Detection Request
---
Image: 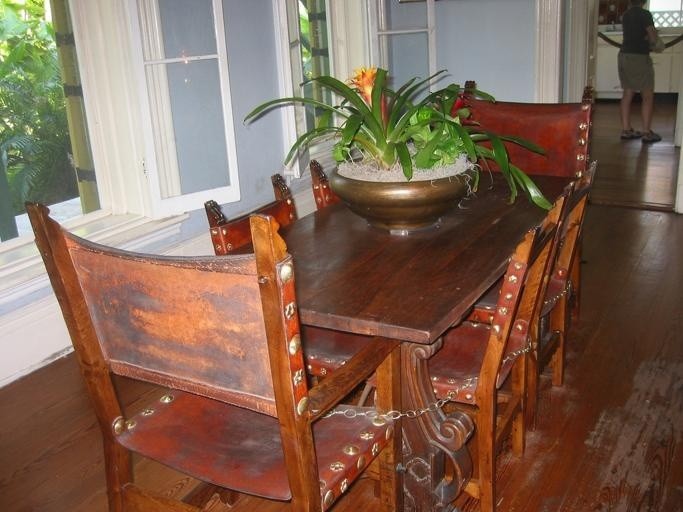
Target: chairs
[457,80,596,178]
[203,174,373,389]
[308,160,341,209]
[466,158,601,388]
[24,201,406,512]
[366,189,570,511]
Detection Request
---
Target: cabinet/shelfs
[594,24,683,94]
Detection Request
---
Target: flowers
[243,63,556,211]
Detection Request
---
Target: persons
[617,0,663,142]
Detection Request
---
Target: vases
[329,155,474,235]
[329,163,480,233]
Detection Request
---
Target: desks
[226,170,578,511]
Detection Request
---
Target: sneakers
[621,129,643,139]
[641,131,661,142]
